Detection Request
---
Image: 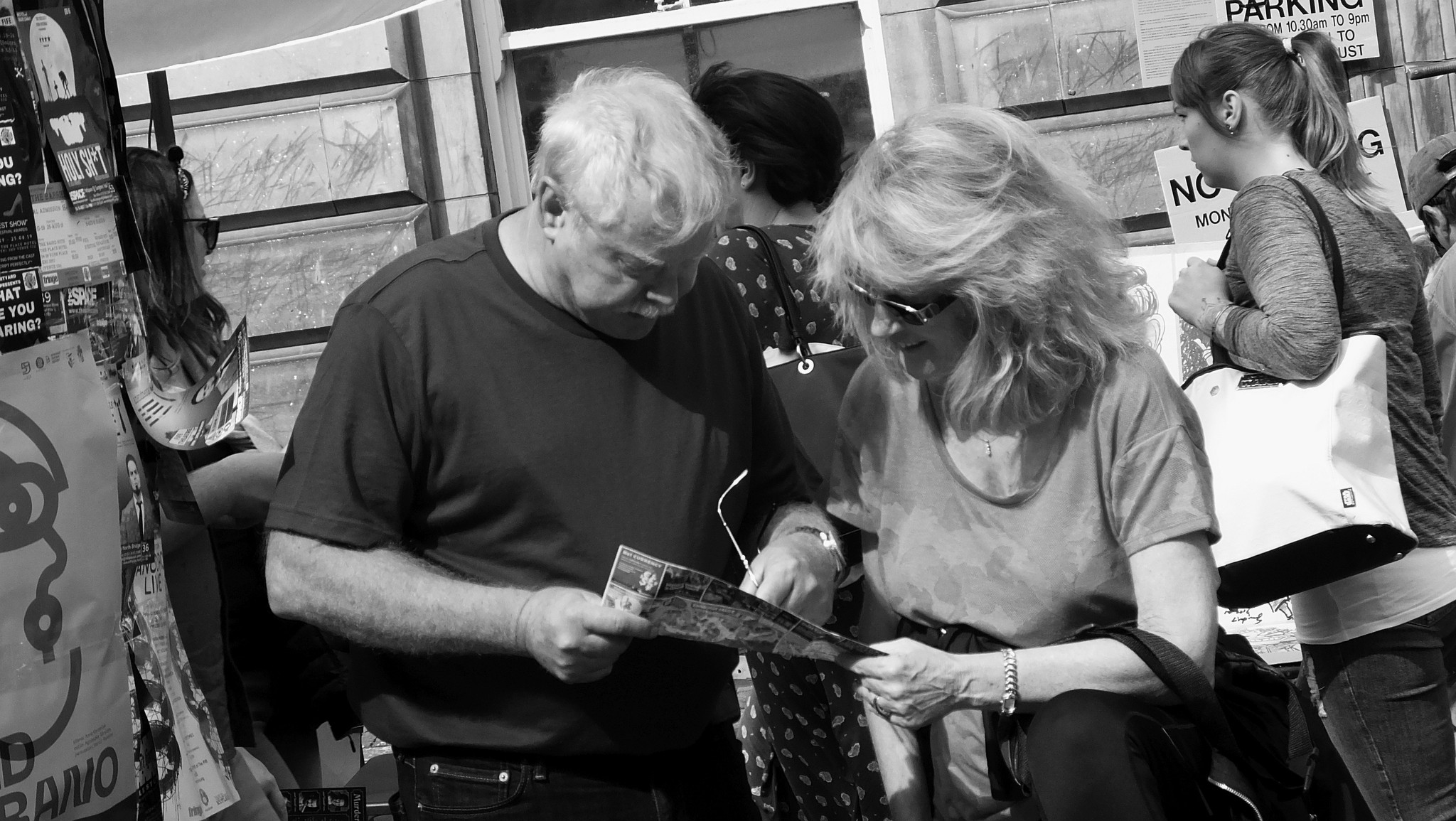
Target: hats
[1407,132,1456,219]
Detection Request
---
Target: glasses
[184,217,220,250]
[845,278,959,327]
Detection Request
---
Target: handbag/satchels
[1183,178,1418,597]
[726,222,870,521]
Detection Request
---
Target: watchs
[780,522,850,587]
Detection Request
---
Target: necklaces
[963,420,1003,458]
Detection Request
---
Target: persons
[689,62,903,821]
[1169,17,1456,821]
[805,104,1223,821]
[259,65,841,820]
[109,142,365,821]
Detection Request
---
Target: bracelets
[1000,646,1020,716]
[1209,302,1235,339]
